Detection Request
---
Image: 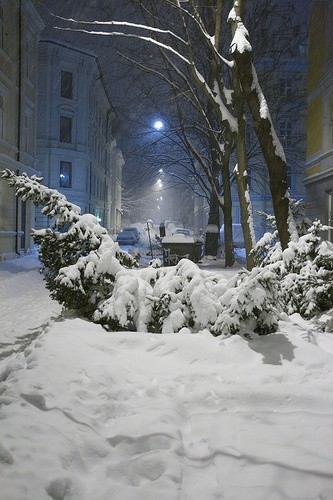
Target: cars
[173,228,193,236]
[116,227,140,245]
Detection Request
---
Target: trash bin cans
[194,240,203,264]
[204,231,219,260]
[161,242,195,267]
